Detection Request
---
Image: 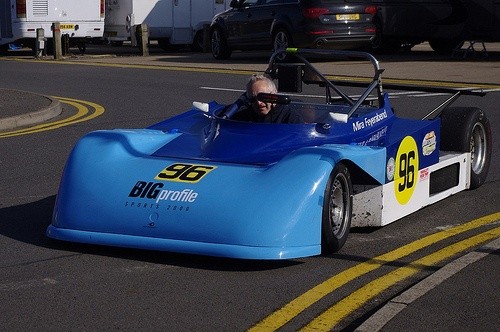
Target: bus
[0,0,107,52]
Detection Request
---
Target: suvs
[211,0,376,57]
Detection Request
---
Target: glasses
[247,95,259,103]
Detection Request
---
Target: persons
[220,73,307,125]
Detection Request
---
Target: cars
[49,47,492,265]
